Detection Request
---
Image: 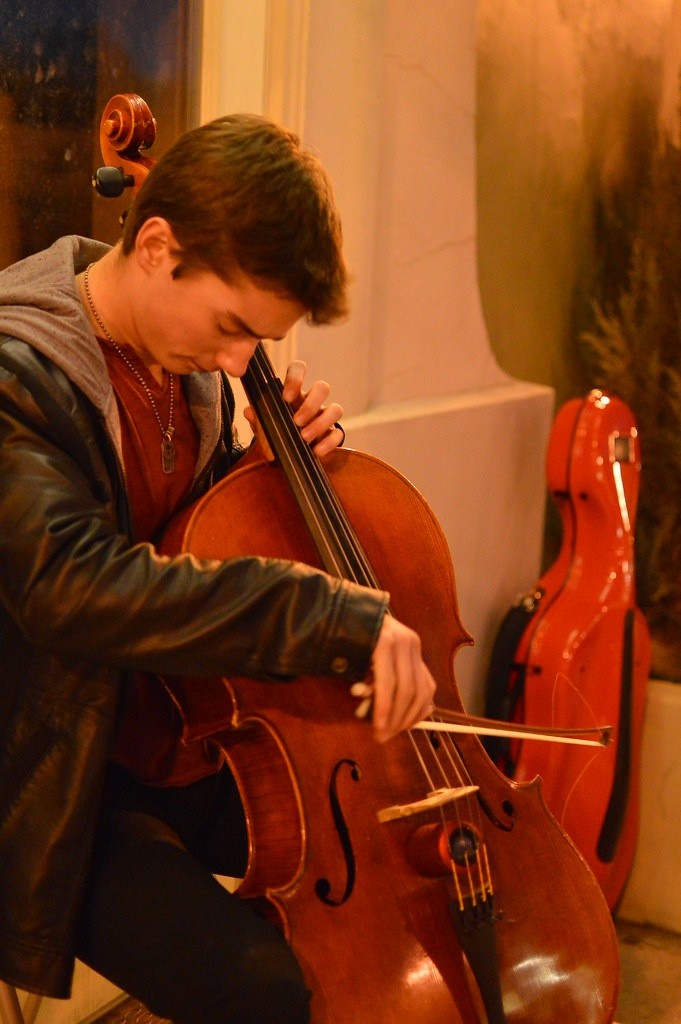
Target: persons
[0,114,437,1024]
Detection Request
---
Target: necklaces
[86,263,176,473]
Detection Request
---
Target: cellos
[92,87,627,1024]
